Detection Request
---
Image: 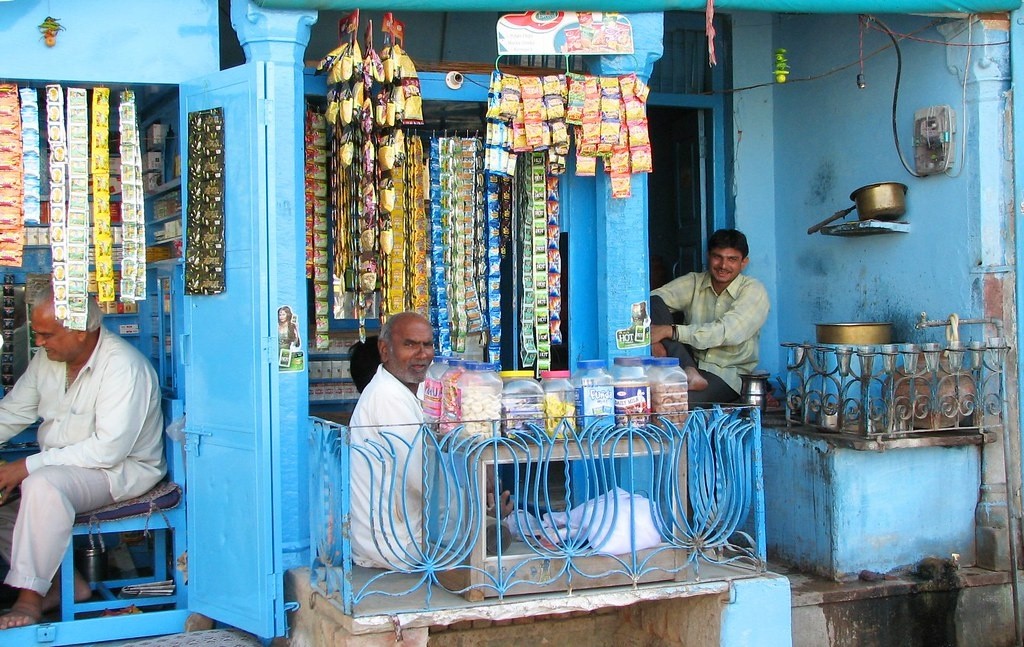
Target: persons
[278,306,300,352]
[343,312,513,572]
[649,228,771,411]
[631,303,648,330]
[350,335,560,520]
[0,283,167,629]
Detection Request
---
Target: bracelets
[670,323,678,342]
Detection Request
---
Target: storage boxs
[89,158,137,313]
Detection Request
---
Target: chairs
[60,398,188,621]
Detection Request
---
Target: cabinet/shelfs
[420,429,689,603]
[308,318,381,404]
[89,154,139,317]
[143,177,182,263]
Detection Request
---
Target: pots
[807,180,905,235]
[813,321,893,345]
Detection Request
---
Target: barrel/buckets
[74,543,109,582]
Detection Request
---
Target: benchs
[283,553,791,647]
